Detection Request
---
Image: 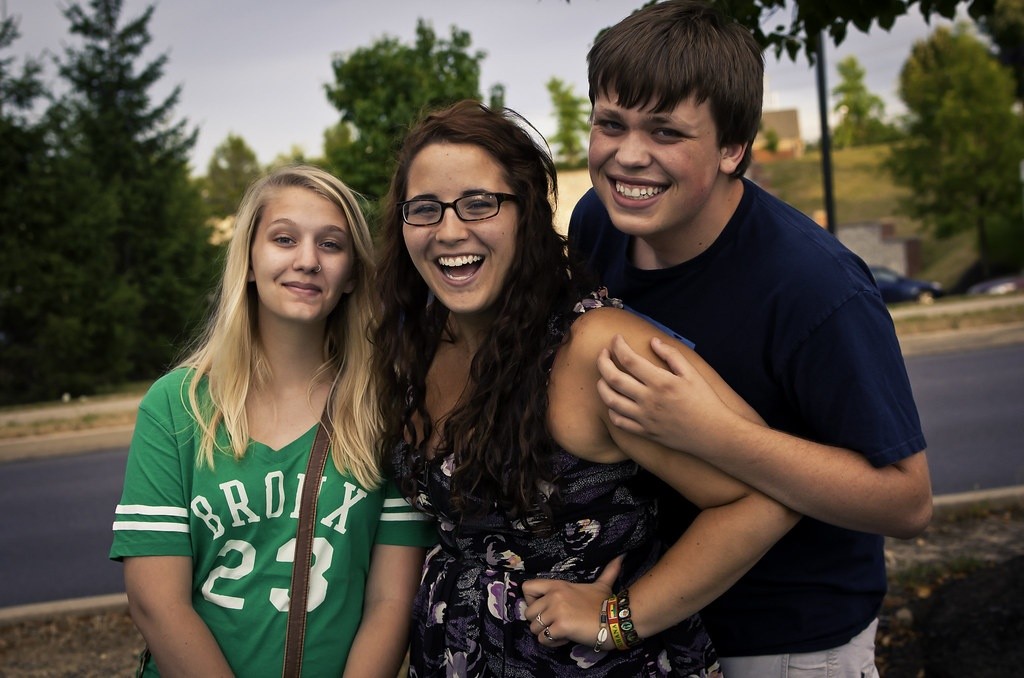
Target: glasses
[388,188,525,229]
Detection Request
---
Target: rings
[536,612,547,627]
[544,628,555,641]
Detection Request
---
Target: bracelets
[593,589,644,652]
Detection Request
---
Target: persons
[108,169,428,678]
[565,5,932,678]
[371,100,796,678]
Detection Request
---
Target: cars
[868,262,942,305]
